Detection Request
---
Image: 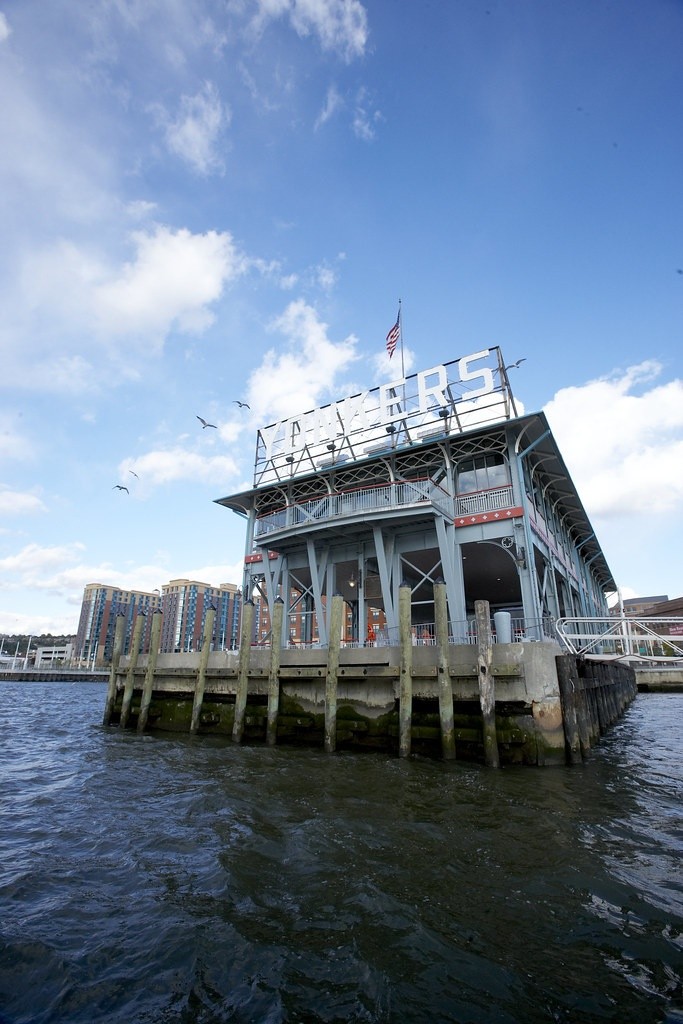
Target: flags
[385,299,401,360]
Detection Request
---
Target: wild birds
[504,358,527,372]
[111,485,129,495]
[231,400,251,410]
[195,415,218,429]
[127,469,140,481]
